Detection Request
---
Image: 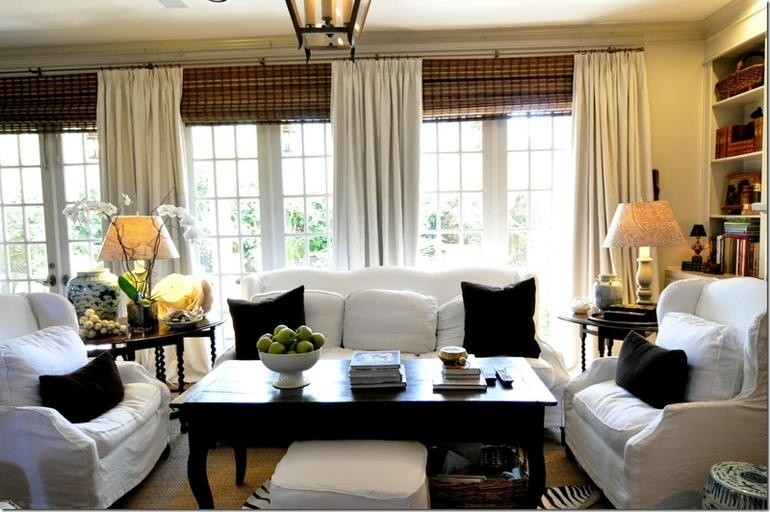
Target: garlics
[79,309,127,339]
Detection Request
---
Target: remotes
[493,365,514,383]
[481,366,497,381]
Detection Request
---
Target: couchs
[272,439,431,511]
[234,272,566,442]
[2,293,175,510]
[568,276,767,512]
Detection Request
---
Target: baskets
[714,48,764,101]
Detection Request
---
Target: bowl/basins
[480,446,514,475]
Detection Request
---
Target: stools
[701,461,768,511]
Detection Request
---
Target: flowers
[63,193,203,302]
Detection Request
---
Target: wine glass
[258,348,321,390]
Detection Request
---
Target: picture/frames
[720,172,762,211]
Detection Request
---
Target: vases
[66,269,119,337]
[127,301,155,331]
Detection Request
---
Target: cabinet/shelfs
[701,31,768,277]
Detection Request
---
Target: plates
[162,318,204,332]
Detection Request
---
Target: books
[433,354,487,390]
[715,218,761,276]
[348,350,407,389]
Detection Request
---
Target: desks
[79,318,226,436]
[557,306,659,377]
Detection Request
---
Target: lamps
[603,198,688,306]
[286,0,371,62]
[689,224,707,263]
[96,215,182,290]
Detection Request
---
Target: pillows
[1,324,86,405]
[435,293,465,353]
[253,290,345,350]
[460,276,539,359]
[342,288,437,353]
[39,350,121,421]
[225,285,307,359]
[614,324,685,410]
[657,304,739,401]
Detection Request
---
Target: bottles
[740,176,761,215]
